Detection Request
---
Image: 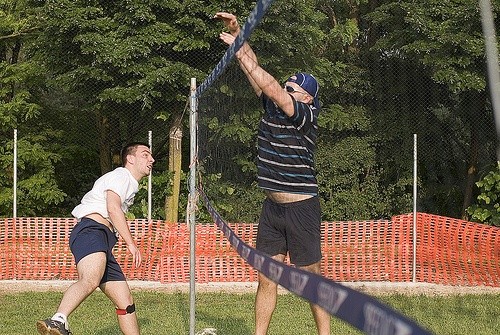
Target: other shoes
[37,318,73,335]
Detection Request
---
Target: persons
[213,12,331,335]
[37,142,155,335]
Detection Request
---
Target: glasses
[282,85,306,95]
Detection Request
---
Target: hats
[287,73,320,109]
